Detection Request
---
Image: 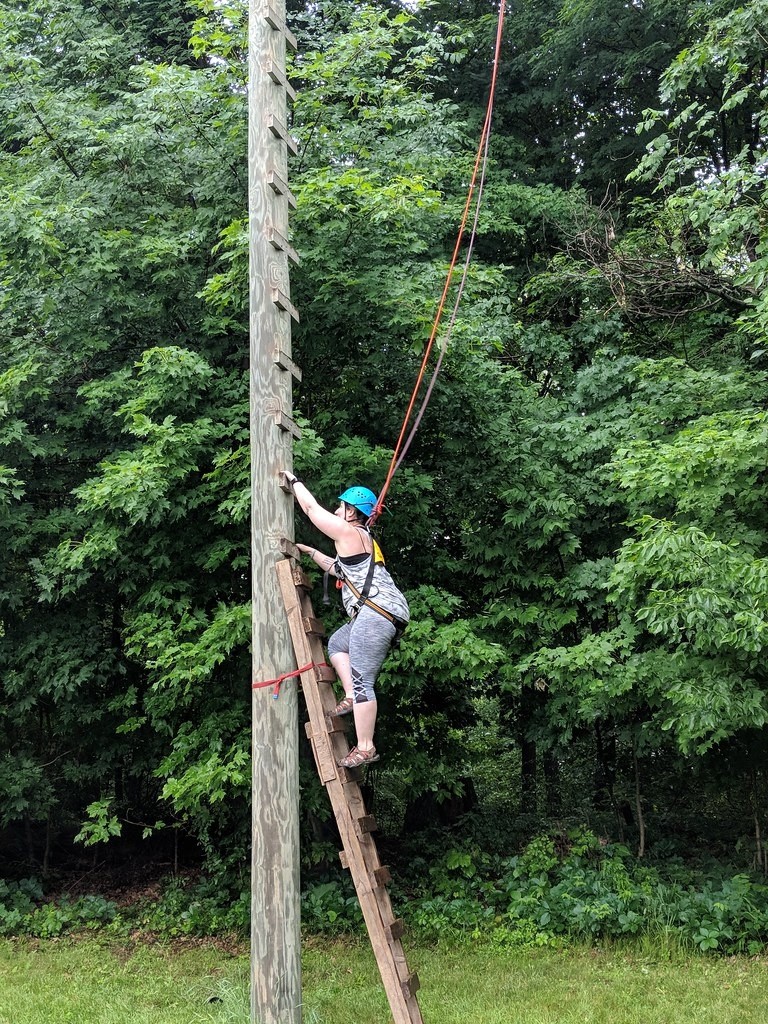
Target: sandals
[326,698,353,717]
[336,746,380,769]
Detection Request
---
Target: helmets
[338,486,377,518]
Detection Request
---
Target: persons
[279,471,410,768]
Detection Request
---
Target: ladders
[276,560,423,1024]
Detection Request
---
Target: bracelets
[311,550,316,560]
[291,478,304,485]
